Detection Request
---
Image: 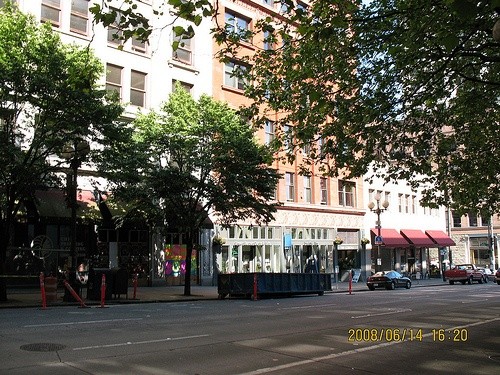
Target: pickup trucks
[446,263,486,285]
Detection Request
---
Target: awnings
[32,188,214,230]
[370,228,456,247]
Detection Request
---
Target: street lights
[369,193,389,273]
[57,141,90,303]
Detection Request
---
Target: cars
[367,270,411,290]
[427,265,438,278]
[494,268,500,285]
[478,269,489,283]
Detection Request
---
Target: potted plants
[334,240,343,244]
[214,237,225,255]
[361,239,371,245]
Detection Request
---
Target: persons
[76,261,88,285]
